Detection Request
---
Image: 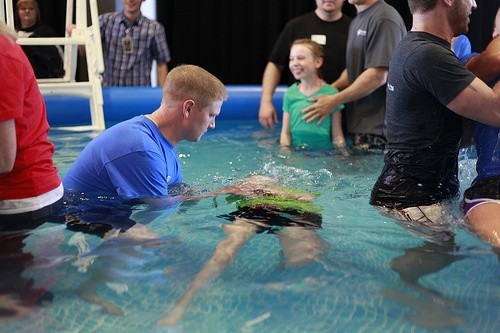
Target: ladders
[0,0,107,131]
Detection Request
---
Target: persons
[369,0,477,329]
[159,175,323,325]
[460,7,500,262]
[66,64,228,315]
[301,0,407,154]
[280,39,349,165]
[258,0,352,144]
[13,0,65,78]
[0,19,63,318]
[68,0,170,87]
